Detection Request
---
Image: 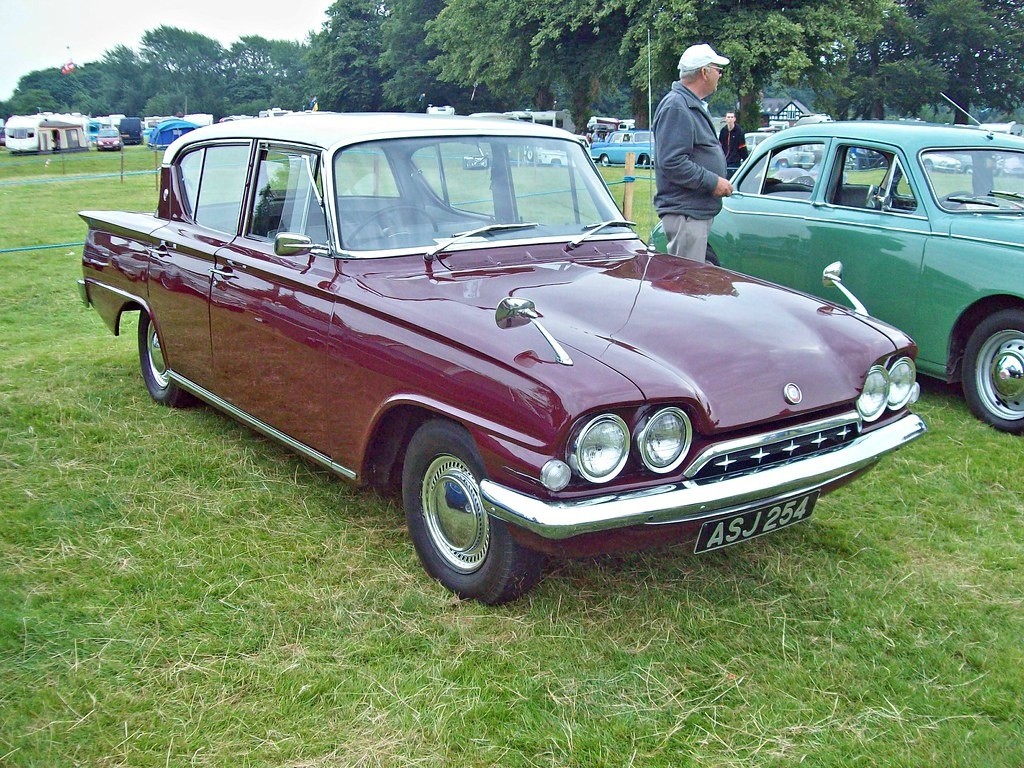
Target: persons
[580,129,610,144]
[651,43,734,263]
[719,110,749,181]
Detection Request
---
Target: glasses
[700,66,723,76]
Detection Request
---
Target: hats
[678,44,730,71]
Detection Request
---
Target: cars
[79,109,926,607]
[0,103,1024,172]
[647,116,1024,436]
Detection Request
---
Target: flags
[309,96,318,111]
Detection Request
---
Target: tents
[146,119,202,150]
